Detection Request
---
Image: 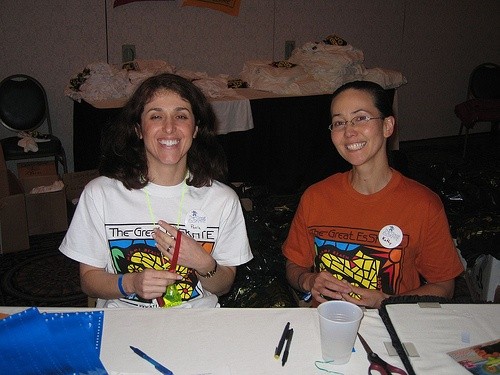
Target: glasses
[328,115,385,133]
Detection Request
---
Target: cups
[317,300,363,365]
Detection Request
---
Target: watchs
[196,261,218,278]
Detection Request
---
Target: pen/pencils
[281,329,293,366]
[130,346,172,375]
[274,322,290,358]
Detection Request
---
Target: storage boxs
[0,161,102,255]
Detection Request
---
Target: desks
[0,304,500,375]
[73,88,396,190]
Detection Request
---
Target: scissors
[357,332,408,375]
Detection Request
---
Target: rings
[166,246,172,253]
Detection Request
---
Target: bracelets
[118,274,132,297]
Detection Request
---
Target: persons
[58,73,253,308]
[282,80,463,308]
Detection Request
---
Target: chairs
[459,63,500,158]
[0,74,69,174]
